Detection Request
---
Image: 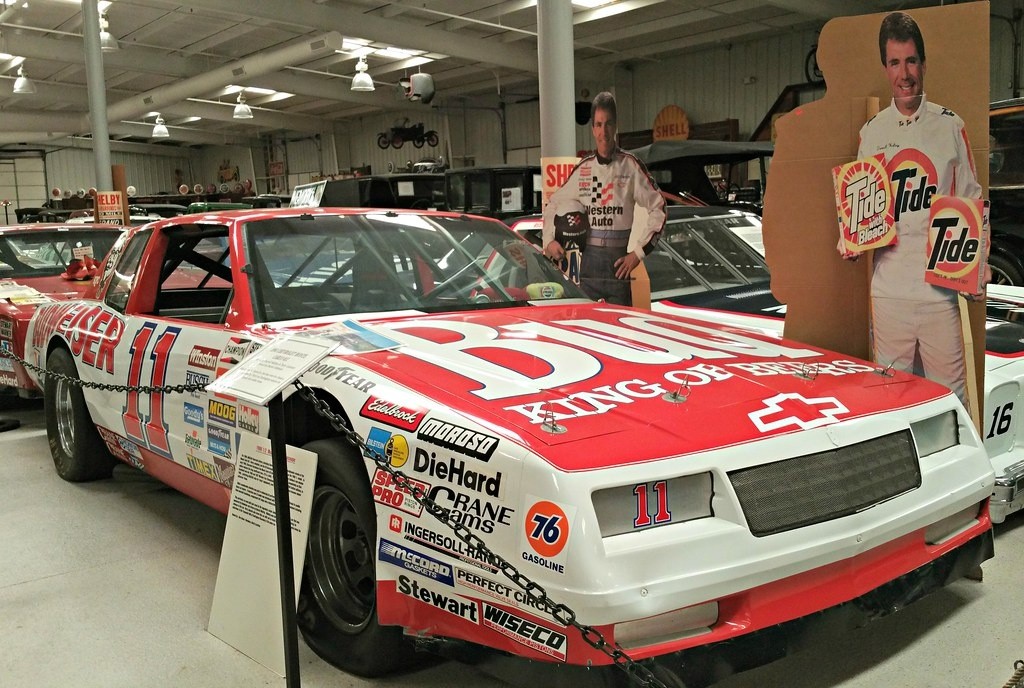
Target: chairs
[350,249,405,311]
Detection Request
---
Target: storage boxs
[832,152,897,256]
[924,194,991,296]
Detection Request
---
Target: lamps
[151,117,169,138]
[98,14,119,52]
[233,93,254,118]
[350,61,375,92]
[13,65,37,94]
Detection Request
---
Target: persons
[542,91,668,307]
[836,13,990,416]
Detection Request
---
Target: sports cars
[21,207,994,681]
[0,193,292,403]
[402,185,1024,556]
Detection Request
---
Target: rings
[624,268,628,271]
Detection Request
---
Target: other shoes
[60,259,90,281]
[84,254,100,278]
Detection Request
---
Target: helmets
[552,199,588,252]
